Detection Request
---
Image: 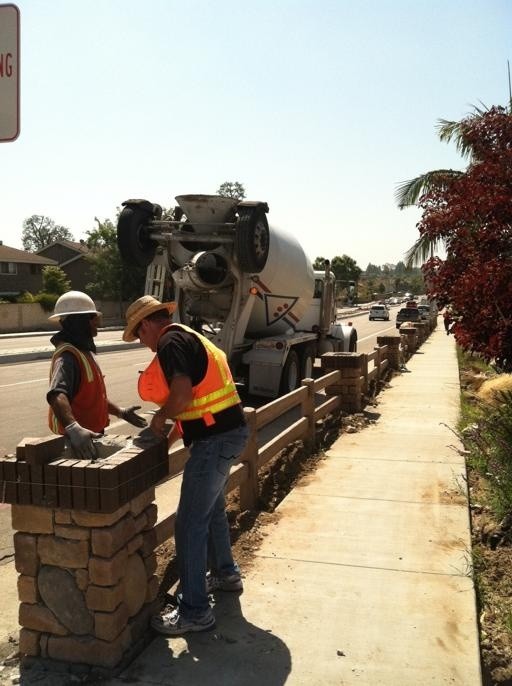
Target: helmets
[48,291,103,322]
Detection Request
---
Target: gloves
[65,420,101,460]
[119,406,148,428]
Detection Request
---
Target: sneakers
[151,608,216,635]
[205,571,243,594]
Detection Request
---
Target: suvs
[395,305,437,329]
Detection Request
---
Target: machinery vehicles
[114,192,361,405]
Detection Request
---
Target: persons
[120,294,250,638]
[443,310,450,331]
[41,288,150,460]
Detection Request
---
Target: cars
[378,290,434,317]
[368,303,390,322]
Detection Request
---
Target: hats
[122,295,178,342]
